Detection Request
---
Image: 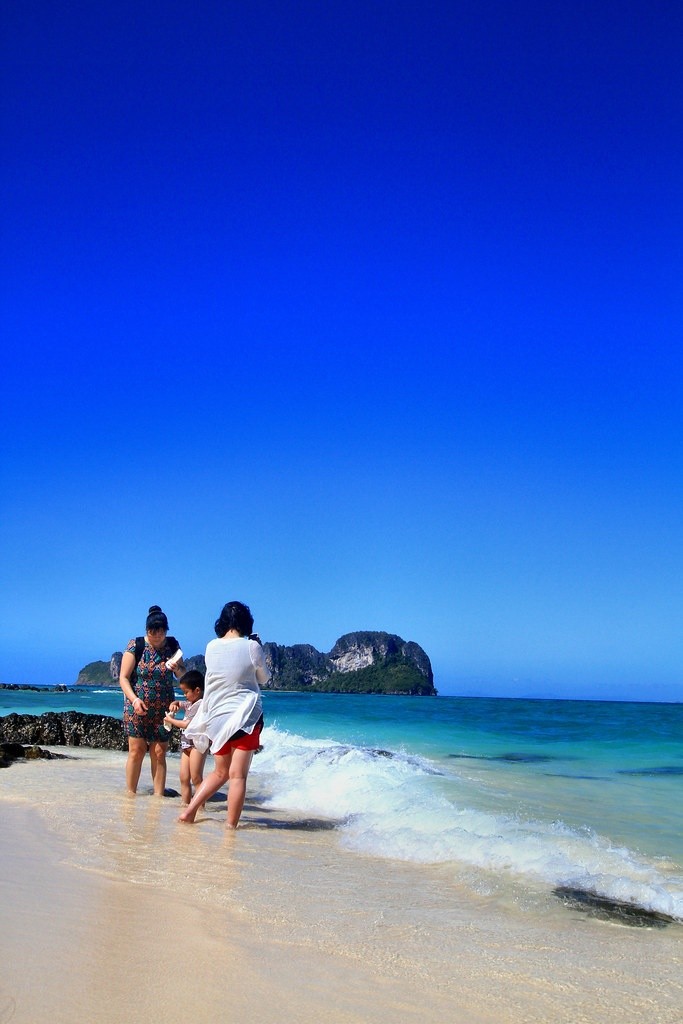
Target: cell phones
[248,633,258,641]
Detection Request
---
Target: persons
[163,670,208,810]
[119,605,186,797]
[178,601,272,828]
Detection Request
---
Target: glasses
[146,631,167,639]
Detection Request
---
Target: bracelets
[132,698,139,706]
